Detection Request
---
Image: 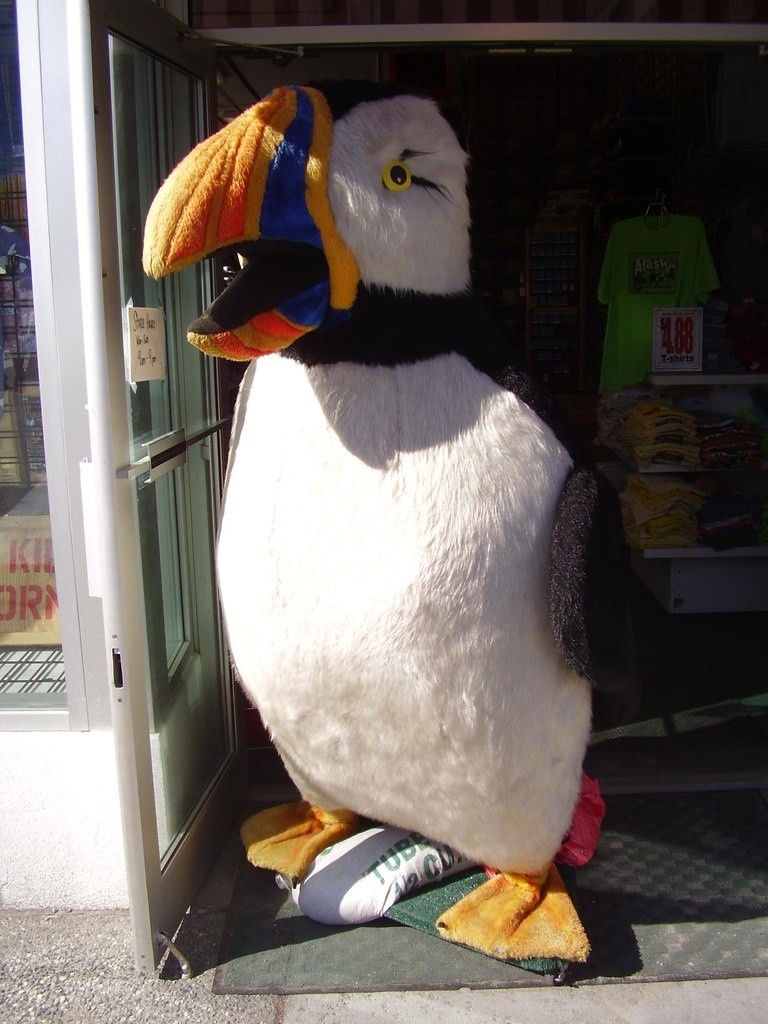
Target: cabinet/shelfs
[522,223,589,392]
[602,373,768,613]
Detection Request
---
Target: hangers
[641,187,670,218]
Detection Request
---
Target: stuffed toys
[141,74,718,966]
